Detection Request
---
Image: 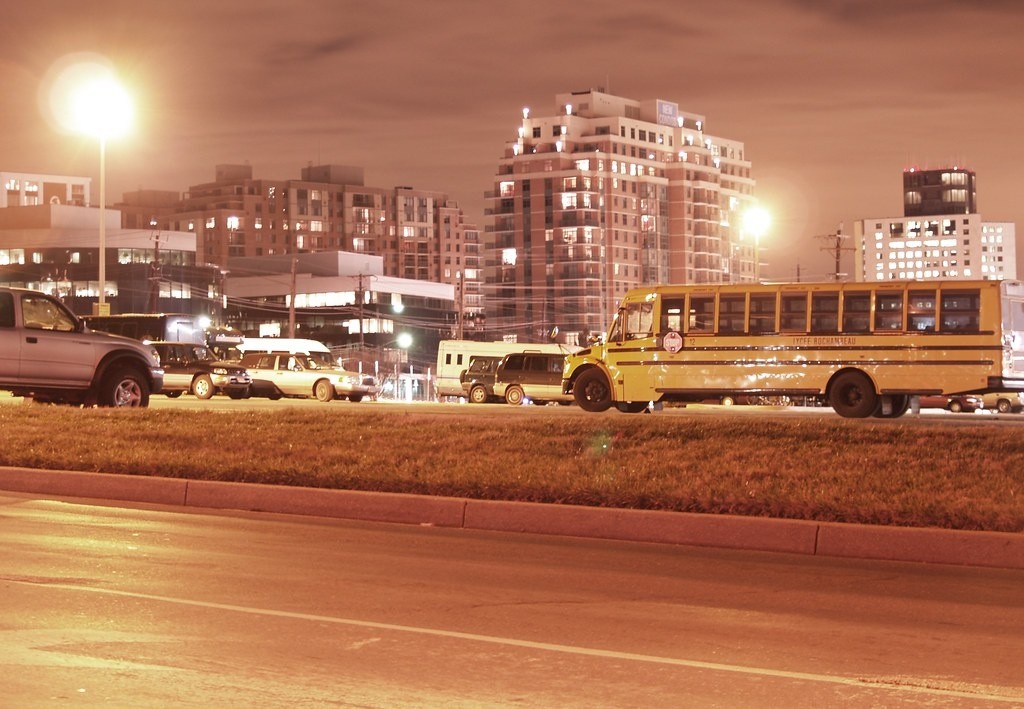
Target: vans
[492,353,580,406]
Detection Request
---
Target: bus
[560,278,1024,418]
[207,335,334,370]
[74,311,206,362]
[432,338,586,403]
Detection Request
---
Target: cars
[971,391,1024,414]
[908,394,979,413]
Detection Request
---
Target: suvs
[0,285,167,413]
[238,351,381,404]
[142,339,252,400]
[459,357,503,404]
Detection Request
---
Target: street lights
[81,86,132,310]
